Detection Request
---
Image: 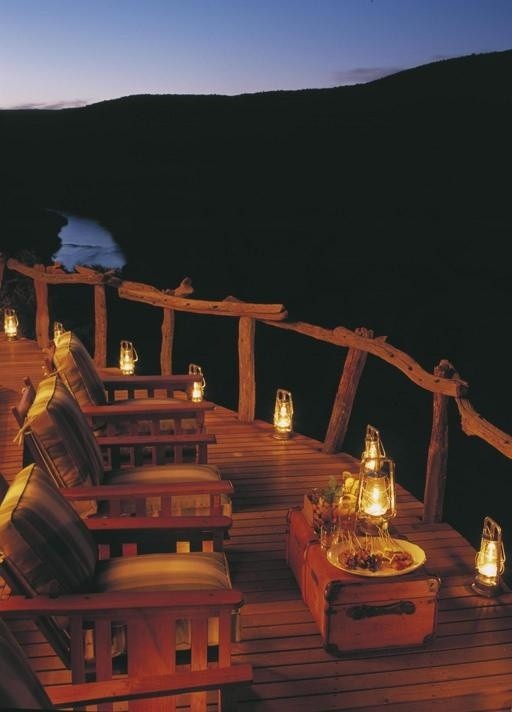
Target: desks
[286,505,443,657]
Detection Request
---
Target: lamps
[470,516,505,597]
[1,305,22,341]
[358,456,395,536]
[119,339,140,375]
[53,321,68,340]
[271,388,296,441]
[189,363,206,403]
[361,424,387,472]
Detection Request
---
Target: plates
[326,537,427,577]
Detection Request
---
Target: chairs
[0,618,252,711]
[11,371,235,548]
[0,463,244,678]
[39,330,216,463]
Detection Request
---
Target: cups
[320,523,340,555]
